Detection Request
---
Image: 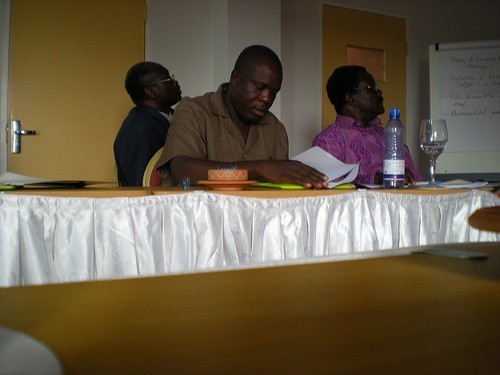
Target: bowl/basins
[207,169,248,180]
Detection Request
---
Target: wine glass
[419,119,448,189]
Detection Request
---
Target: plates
[196,180,257,191]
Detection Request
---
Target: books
[23,181,85,189]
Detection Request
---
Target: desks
[0,183,500,375]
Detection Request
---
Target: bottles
[382,108,406,189]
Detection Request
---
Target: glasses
[152,74,175,85]
[356,84,378,93]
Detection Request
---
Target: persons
[157,44,329,191]
[312,65,425,188]
[113,60,183,187]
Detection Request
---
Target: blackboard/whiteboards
[429,39,500,174]
